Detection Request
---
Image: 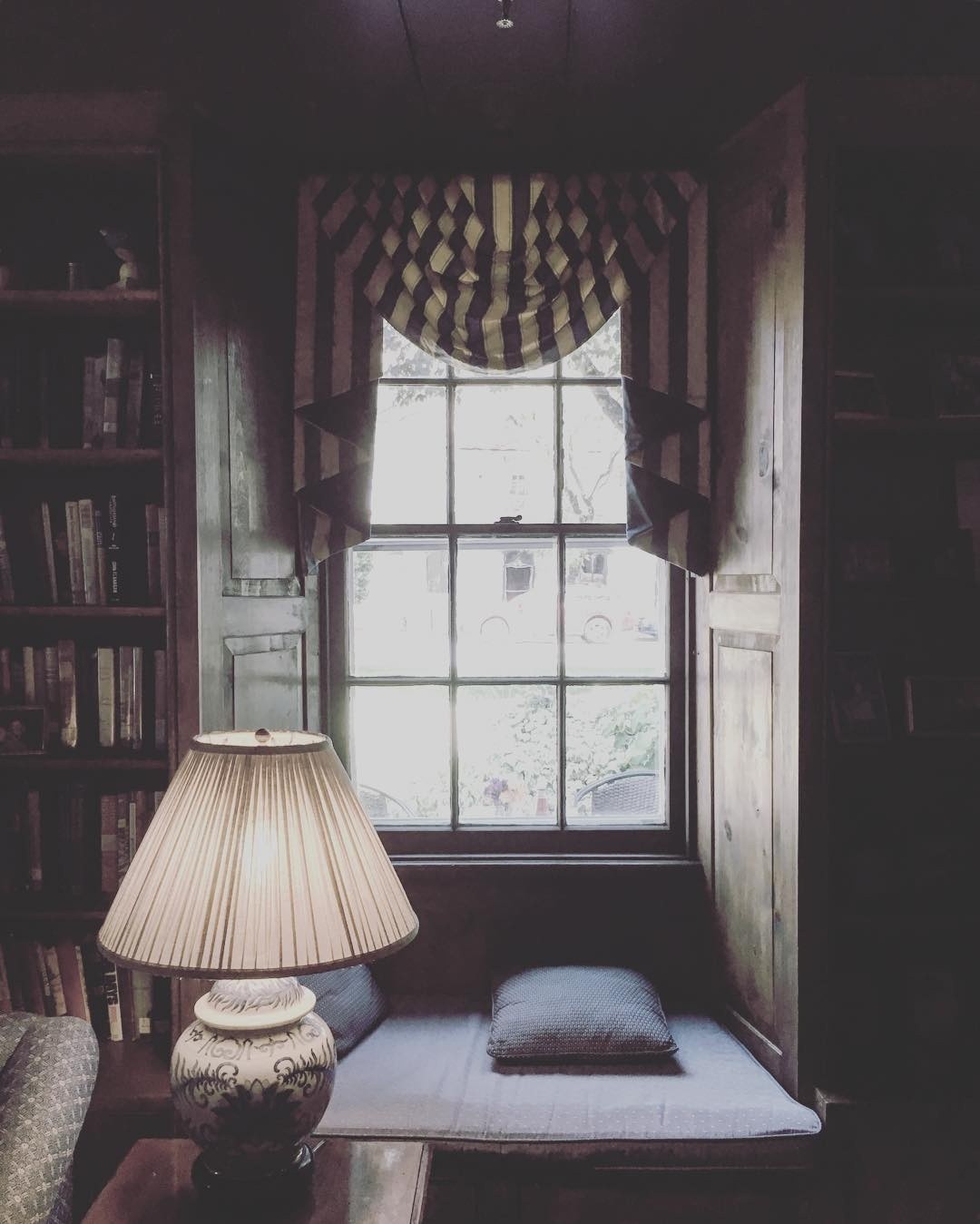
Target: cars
[454,584,634,645]
[638,612,664,641]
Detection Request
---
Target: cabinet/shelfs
[0,86,176,1119]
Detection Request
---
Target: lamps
[97,726,421,1224]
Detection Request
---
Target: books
[2,330,166,1049]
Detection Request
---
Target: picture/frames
[833,365,888,424]
[902,675,980,741]
[926,348,980,422]
[830,650,893,743]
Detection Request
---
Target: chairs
[360,782,415,820]
[574,769,659,821]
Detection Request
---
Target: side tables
[80,1136,432,1224]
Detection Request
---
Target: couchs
[0,1010,101,1224]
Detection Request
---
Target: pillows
[293,961,393,1069]
[484,962,679,1069]
